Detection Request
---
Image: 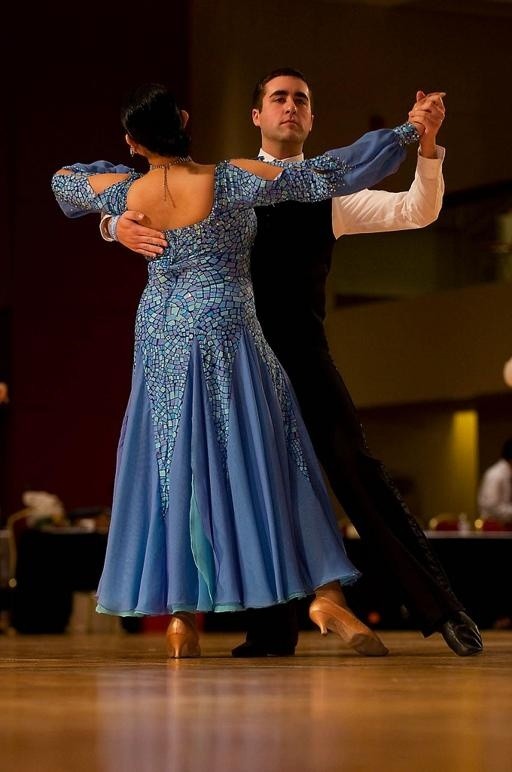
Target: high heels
[309,596,388,656]
[166,616,201,658]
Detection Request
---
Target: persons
[51,88,445,662]
[98,69,485,658]
[475,436,512,532]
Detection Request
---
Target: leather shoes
[231,642,295,657]
[440,609,483,657]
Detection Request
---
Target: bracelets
[108,214,120,243]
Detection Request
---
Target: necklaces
[148,155,193,172]
